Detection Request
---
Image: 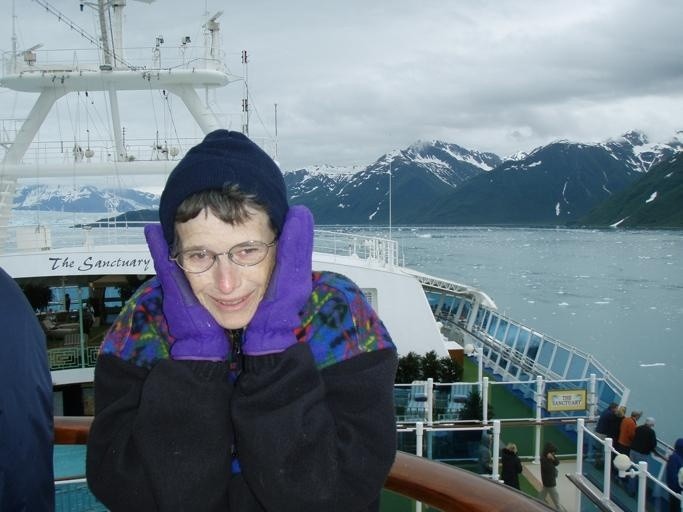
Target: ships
[1,0,683,511]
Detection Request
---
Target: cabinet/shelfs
[444,341,464,367]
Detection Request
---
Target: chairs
[407,380,472,412]
[42,320,80,338]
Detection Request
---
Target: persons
[537,441,562,511]
[84,129,399,512]
[626,416,657,499]
[665,438,683,512]
[607,404,628,473]
[614,409,642,487]
[475,433,494,475]
[593,401,619,471]
[501,442,524,489]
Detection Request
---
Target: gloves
[240,206,314,357]
[145,223,230,359]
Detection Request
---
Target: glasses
[168,237,278,274]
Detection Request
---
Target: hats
[159,129,289,246]
